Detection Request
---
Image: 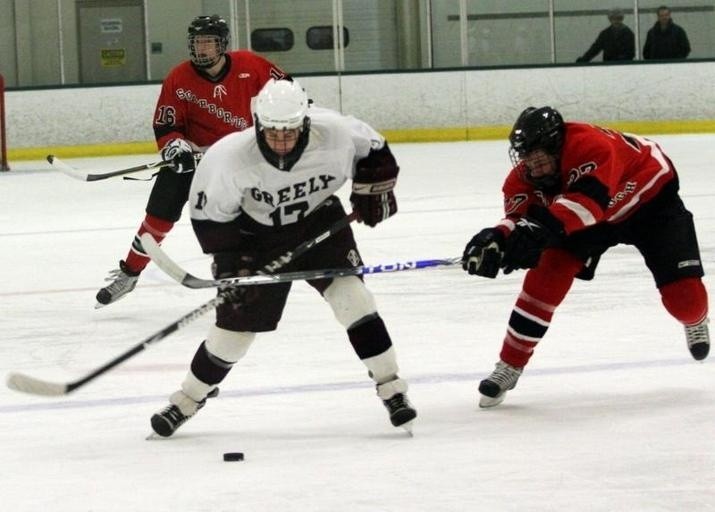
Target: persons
[150,79,421,438]
[93,14,315,305]
[464,106,712,400]
[576,8,635,64]
[642,5,692,60]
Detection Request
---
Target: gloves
[350,168,565,277]
[162,137,198,174]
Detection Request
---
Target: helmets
[255,78,309,157]
[508,106,564,181]
[187,14,231,67]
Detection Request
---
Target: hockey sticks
[45,153,173,182]
[140,231,464,289]
[7,213,355,396]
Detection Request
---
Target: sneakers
[376,378,416,426]
[96,260,139,304]
[479,359,523,395]
[684,320,709,361]
[151,391,205,438]
